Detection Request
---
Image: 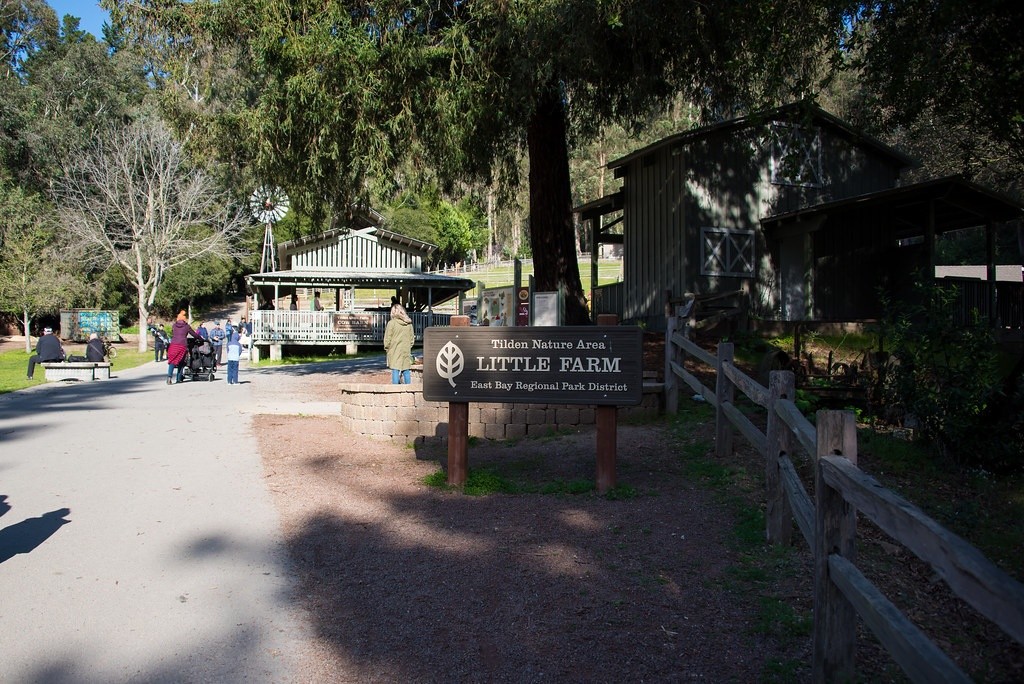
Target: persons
[86,333,107,362]
[227,332,242,385]
[258,297,274,310]
[197,322,208,341]
[155,324,169,362]
[26,326,64,380]
[226,318,258,348]
[482,297,499,316]
[99,331,111,354]
[167,314,204,385]
[391,296,401,308]
[290,294,298,310]
[384,304,414,383]
[210,322,225,366]
[314,292,324,311]
[33,322,59,337]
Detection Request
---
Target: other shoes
[167,377,171,385]
[228,383,231,385]
[178,381,182,383]
[27,376,33,380]
[234,383,240,385]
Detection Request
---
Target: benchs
[41,362,98,381]
[72,362,113,380]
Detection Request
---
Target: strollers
[179,339,220,382]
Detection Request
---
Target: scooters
[103,335,119,359]
